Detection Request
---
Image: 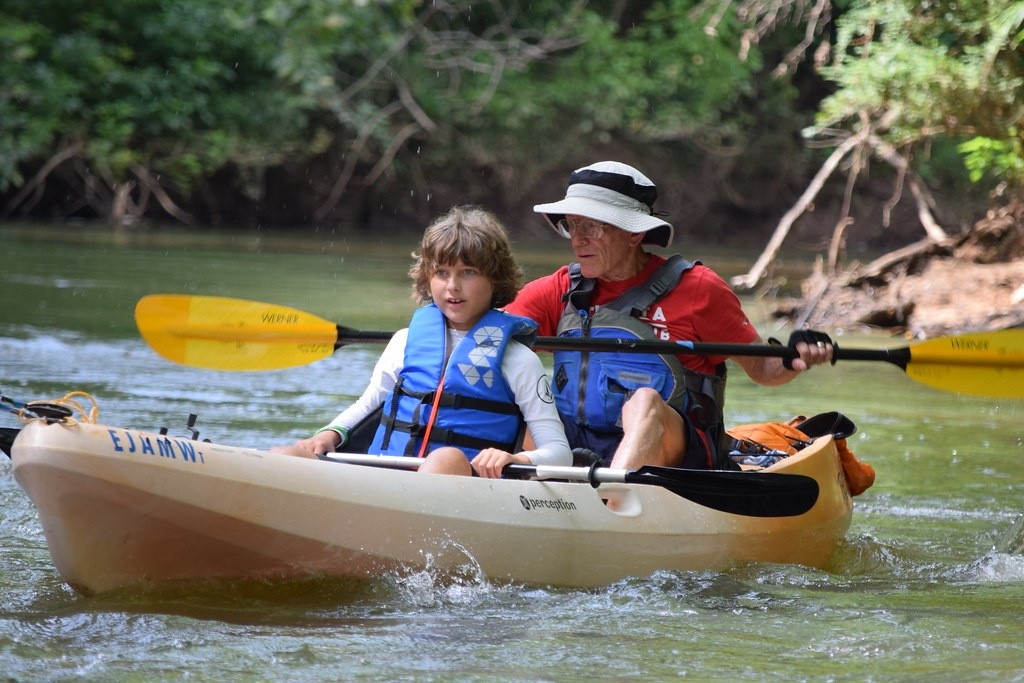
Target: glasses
[554,217,620,240]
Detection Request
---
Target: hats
[532,160,675,252]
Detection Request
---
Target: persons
[268,207,573,480]
[496,161,834,512]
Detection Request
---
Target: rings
[817,341,825,347]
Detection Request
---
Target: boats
[9,417,857,604]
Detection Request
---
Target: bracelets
[314,426,348,448]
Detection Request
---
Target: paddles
[0,425,821,519]
[132,291,1024,413]
